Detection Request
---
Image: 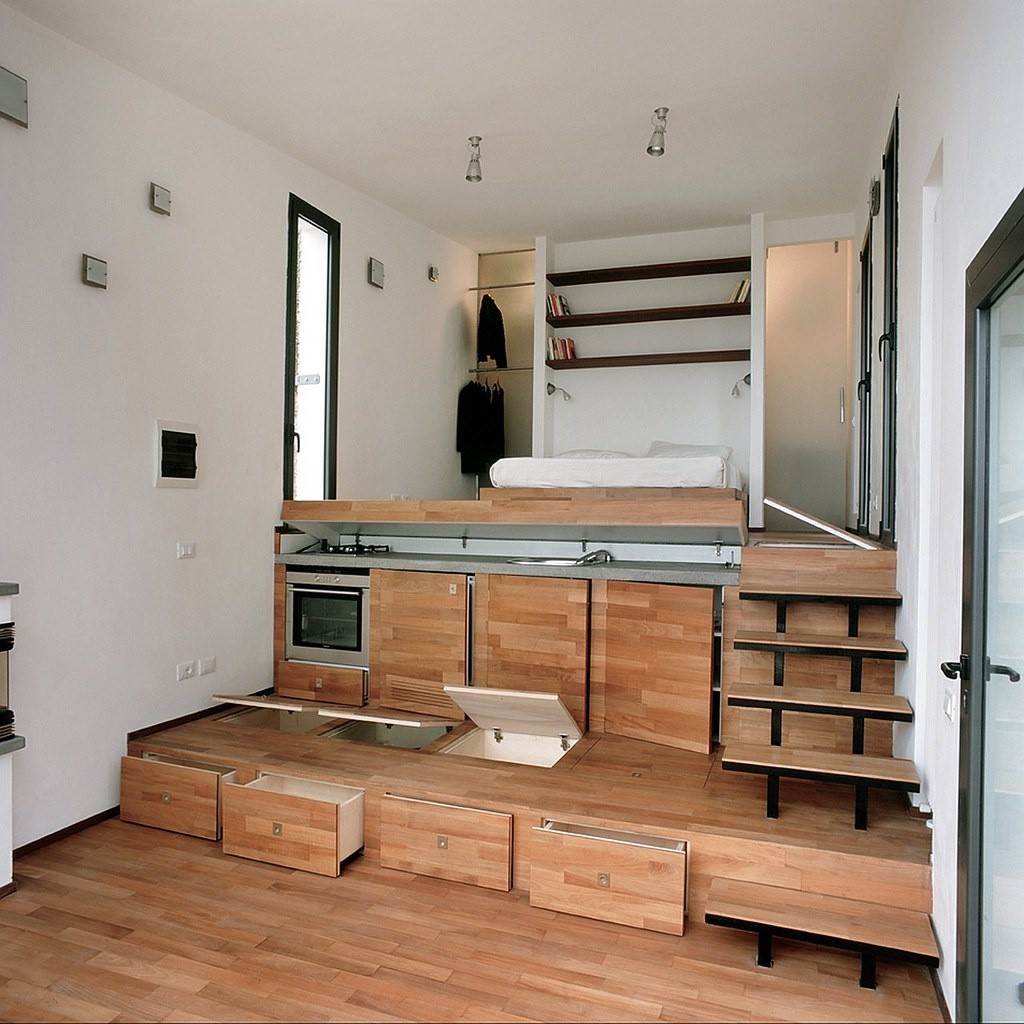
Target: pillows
[553,449,634,457]
[644,442,733,460]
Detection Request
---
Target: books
[730,279,750,303]
[547,293,570,317]
[547,336,576,360]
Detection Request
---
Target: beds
[489,457,746,491]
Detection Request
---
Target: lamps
[732,374,750,395]
[647,108,669,155]
[547,383,571,401]
[465,136,483,182]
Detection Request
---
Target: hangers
[487,286,495,299]
[471,368,501,389]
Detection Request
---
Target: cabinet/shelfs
[121,565,725,935]
[467,282,536,373]
[545,254,751,369]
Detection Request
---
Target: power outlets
[177,660,194,681]
